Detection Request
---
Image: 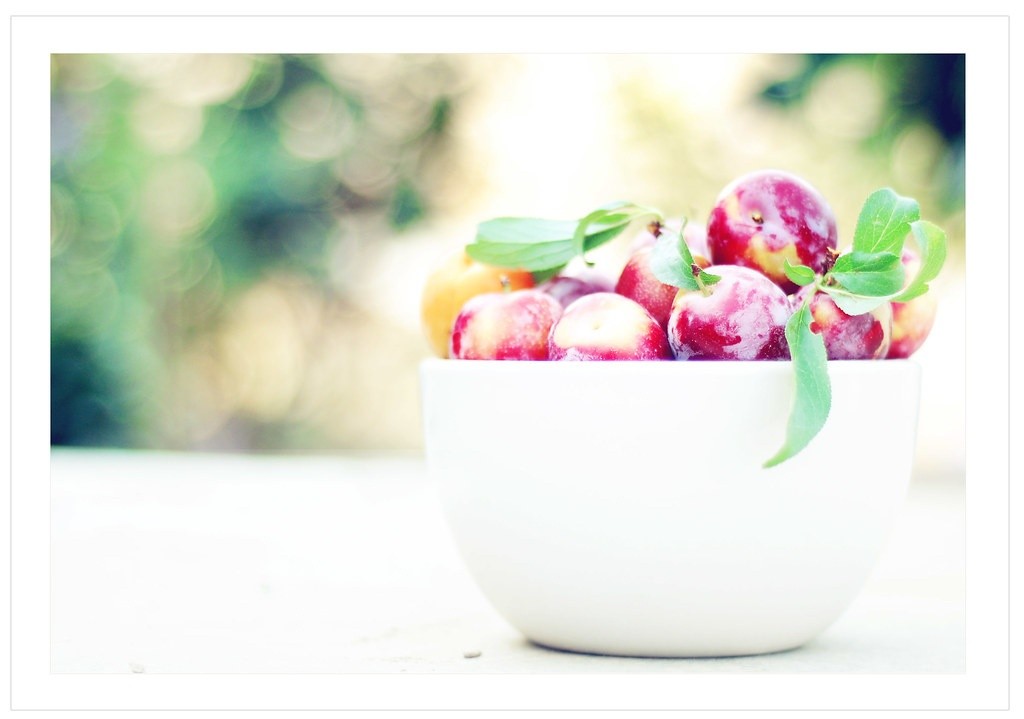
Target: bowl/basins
[421,360,915,660]
